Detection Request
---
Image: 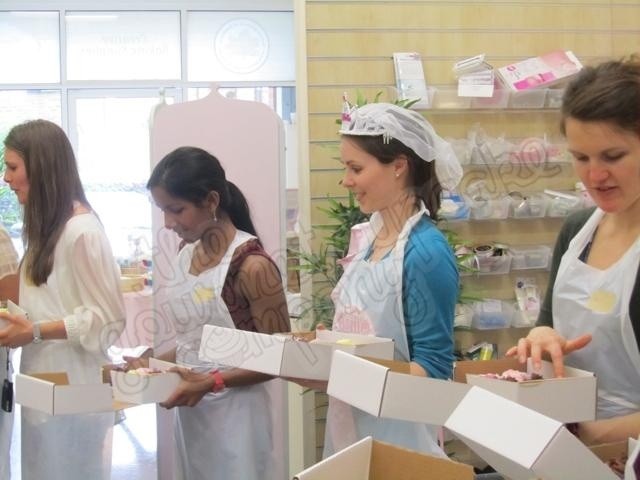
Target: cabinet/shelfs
[389,86,597,330]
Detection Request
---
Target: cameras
[2,379,14,412]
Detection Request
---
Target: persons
[269,100,461,459]
[119,145,293,479]
[1,116,126,480]
[0,226,24,480]
[500,57,640,480]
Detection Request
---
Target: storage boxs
[0,299,620,480]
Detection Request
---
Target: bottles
[479,343,493,361]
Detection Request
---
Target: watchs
[33,322,44,345]
[208,368,225,395]
